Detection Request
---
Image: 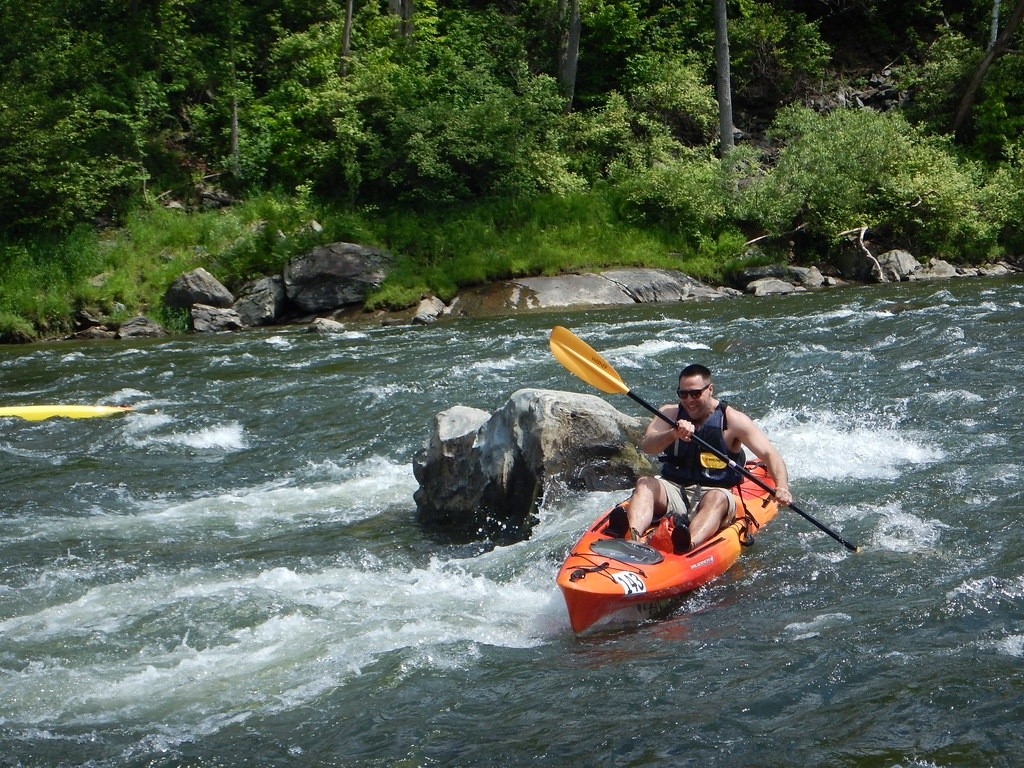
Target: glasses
[676,383,709,399]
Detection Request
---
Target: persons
[609,364,794,555]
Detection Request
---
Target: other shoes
[601,506,630,540]
[671,524,691,555]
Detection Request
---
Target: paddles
[548,324,863,551]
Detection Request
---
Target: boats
[556,455,780,639]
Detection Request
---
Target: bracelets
[774,487,792,497]
